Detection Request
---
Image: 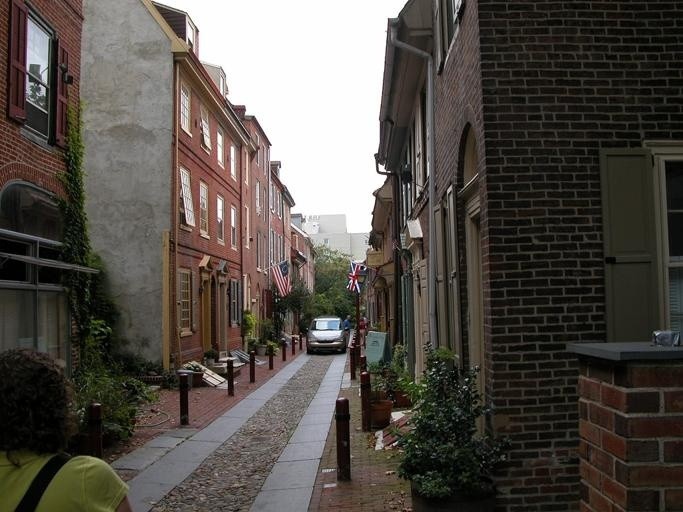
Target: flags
[271,261,291,297]
[345,261,367,294]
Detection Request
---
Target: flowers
[183,360,207,372]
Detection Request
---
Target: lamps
[372,258,394,289]
[375,154,412,182]
[57,61,74,84]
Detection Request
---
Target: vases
[194,373,203,386]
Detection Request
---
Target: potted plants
[256,336,267,356]
[370,345,412,427]
[205,349,218,367]
[386,343,512,512]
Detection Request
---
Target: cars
[305,315,349,354]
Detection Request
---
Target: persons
[0,346,133,511]
[342,314,351,348]
[358,318,367,346]
[359,311,368,324]
